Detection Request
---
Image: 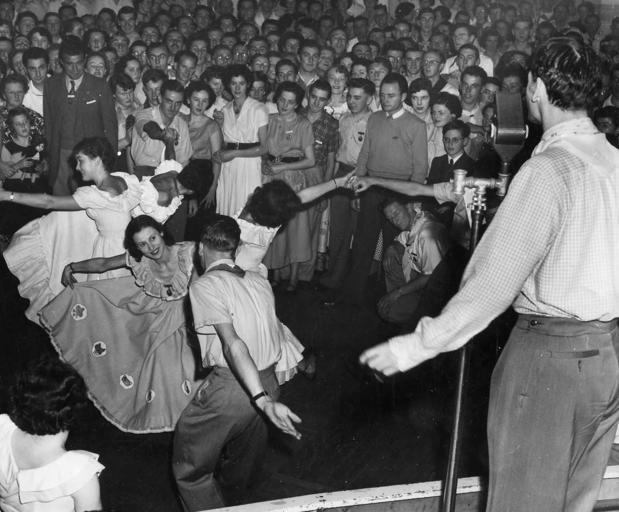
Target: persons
[141,25,160,46]
[147,41,169,71]
[0,19,13,41]
[337,53,358,72]
[182,79,224,240]
[420,50,446,92]
[218,14,237,33]
[1,357,106,512]
[478,76,500,106]
[423,121,479,214]
[44,13,62,42]
[118,6,138,33]
[205,28,224,50]
[1,73,44,140]
[327,28,346,54]
[366,59,391,113]
[367,27,385,46]
[332,0,392,37]
[111,34,130,55]
[236,21,259,42]
[592,18,619,149]
[427,93,463,173]
[136,0,195,18]
[442,25,494,77]
[130,41,148,67]
[0,38,15,67]
[392,0,435,47]
[58,6,77,21]
[43,35,118,196]
[429,0,455,50]
[221,33,238,47]
[348,73,428,305]
[153,12,172,36]
[257,80,315,293]
[319,47,336,71]
[0,1,16,20]
[296,40,319,87]
[414,227,473,323]
[501,70,525,93]
[101,46,119,73]
[16,11,37,32]
[46,42,66,73]
[85,51,111,79]
[279,32,304,56]
[248,36,269,55]
[267,52,282,79]
[1,136,146,282]
[2,128,213,334]
[360,37,619,512]
[250,72,279,114]
[321,79,376,293]
[453,0,532,25]
[30,27,52,49]
[296,0,333,39]
[175,16,194,39]
[323,64,351,121]
[351,43,373,61]
[118,55,141,84]
[265,32,280,51]
[459,66,485,125]
[251,54,269,73]
[274,58,297,84]
[232,41,249,65]
[350,175,478,227]
[109,74,141,172]
[4,109,48,193]
[237,0,255,20]
[173,50,197,83]
[346,16,369,53]
[532,0,600,40]
[12,35,31,49]
[131,79,193,180]
[192,6,211,29]
[233,167,360,279]
[408,79,435,138]
[62,18,85,40]
[80,14,98,32]
[378,198,448,323]
[213,45,233,66]
[351,59,369,79]
[255,0,296,34]
[10,48,28,74]
[38,216,214,433]
[166,30,187,53]
[369,41,380,60]
[385,43,404,73]
[299,80,340,289]
[21,47,55,118]
[214,65,269,216]
[186,34,212,80]
[495,15,532,70]
[171,214,301,512]
[85,30,108,50]
[401,48,424,85]
[98,8,116,31]
[220,0,234,15]
[446,44,480,89]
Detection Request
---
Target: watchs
[249,391,269,404]
[10,191,14,201]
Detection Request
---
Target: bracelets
[332,178,338,190]
[69,262,76,274]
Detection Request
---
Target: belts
[226,143,261,150]
[266,153,298,163]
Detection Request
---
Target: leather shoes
[271,281,282,289]
[286,284,300,295]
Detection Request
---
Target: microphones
[490,88,530,161]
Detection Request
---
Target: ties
[68,81,77,95]
[449,158,455,164]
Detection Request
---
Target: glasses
[235,53,249,57]
[130,51,147,56]
[150,54,169,61]
[423,61,441,67]
[301,52,321,61]
[389,56,402,62]
[217,55,231,59]
